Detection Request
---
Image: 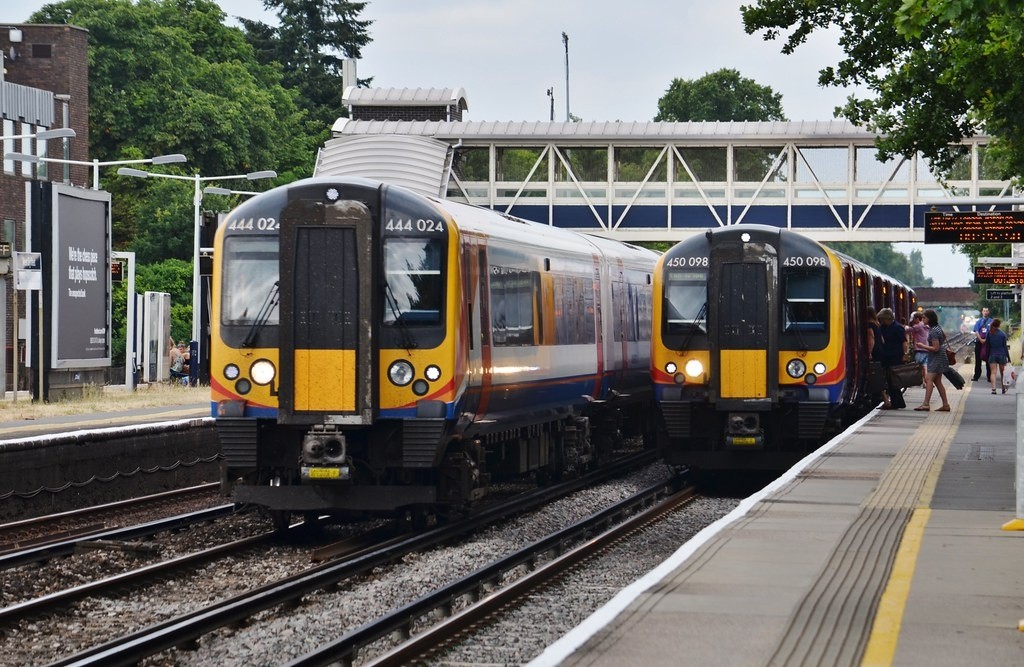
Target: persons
[167,338,190,381]
[864,306,1011,413]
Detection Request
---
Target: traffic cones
[117,166,278,389]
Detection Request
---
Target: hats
[876,307,894,319]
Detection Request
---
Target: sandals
[934,406,950,411]
[914,405,929,411]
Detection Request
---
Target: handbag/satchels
[1002,362,1015,386]
[980,343,988,361]
[946,349,956,365]
[890,360,922,392]
[985,332,990,355]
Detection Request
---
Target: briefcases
[857,353,889,393]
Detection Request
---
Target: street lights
[5,147,187,191]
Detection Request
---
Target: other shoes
[991,388,996,394]
[923,382,935,387]
[891,400,906,409]
[883,401,891,409]
[988,378,991,382]
[1002,387,1008,394]
[971,377,978,381]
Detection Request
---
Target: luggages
[943,367,965,389]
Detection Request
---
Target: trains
[644,217,918,490]
[197,173,705,537]
